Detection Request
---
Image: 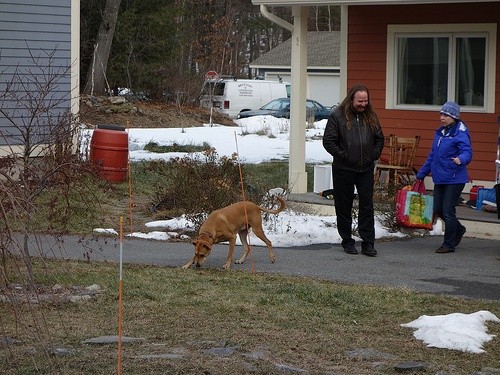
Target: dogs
[182,195,288,269]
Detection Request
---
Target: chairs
[374,134,421,192]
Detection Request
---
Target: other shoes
[361,243,377,256]
[454,225,466,247]
[435,243,455,253]
[344,244,357,254]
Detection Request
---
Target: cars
[236,97,334,122]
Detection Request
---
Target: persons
[322,85,385,256]
[417,101,472,253]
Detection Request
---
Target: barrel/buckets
[89,124,129,184]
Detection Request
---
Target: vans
[199,78,292,119]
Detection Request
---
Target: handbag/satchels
[394,179,434,230]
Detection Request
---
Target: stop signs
[203,70,218,81]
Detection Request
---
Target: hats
[440,101,460,119]
